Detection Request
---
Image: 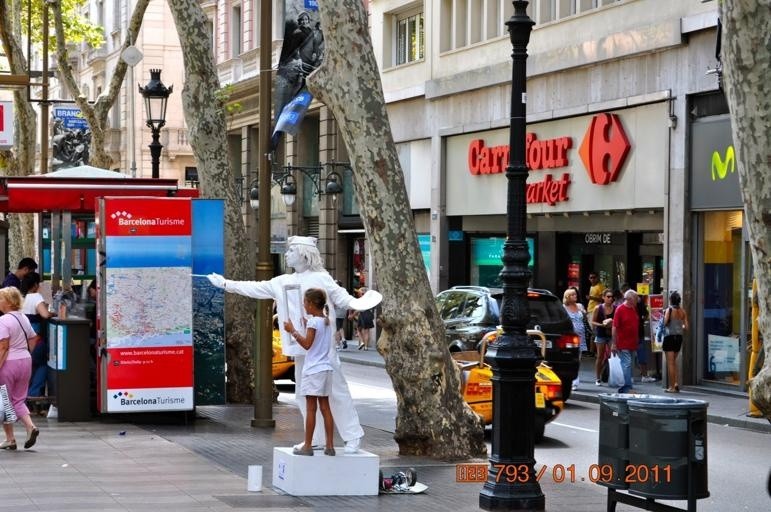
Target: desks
[45,316,89,422]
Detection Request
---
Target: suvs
[436,284,583,402]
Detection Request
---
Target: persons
[205,235,384,455]
[334,279,352,352]
[20,272,58,397]
[87,278,96,365]
[0,257,38,290]
[284,287,338,457]
[0,285,40,451]
[348,286,375,351]
[561,271,690,393]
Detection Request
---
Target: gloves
[207,271,227,290]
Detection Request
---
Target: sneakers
[292,446,314,455]
[344,436,362,455]
[323,447,337,456]
[641,375,658,384]
[595,379,602,386]
[293,438,327,451]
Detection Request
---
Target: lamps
[280,162,324,206]
[667,115,677,129]
[326,158,352,199]
[249,168,282,208]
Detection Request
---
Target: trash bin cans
[595,392,710,511]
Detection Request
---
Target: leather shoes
[24,428,40,449]
[0,442,18,449]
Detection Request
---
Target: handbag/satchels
[1,384,18,425]
[607,351,625,388]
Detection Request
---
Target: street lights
[137,70,175,178]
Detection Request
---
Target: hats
[284,233,321,248]
